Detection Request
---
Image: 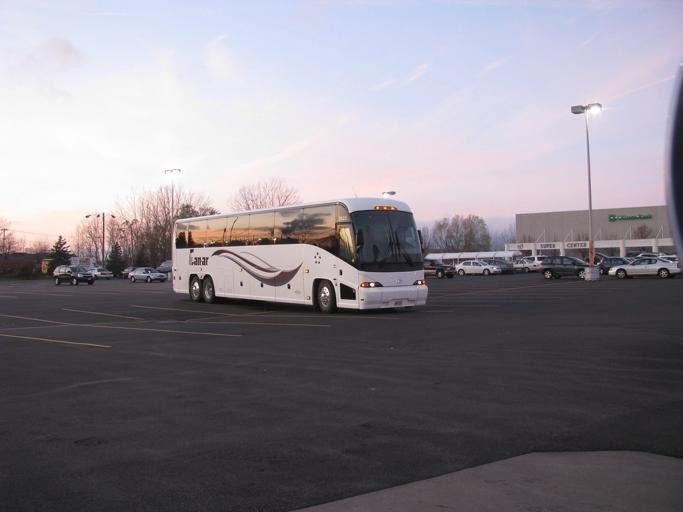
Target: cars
[484,249,677,275]
[125,266,167,283]
[453,259,503,277]
[119,266,136,279]
[607,256,680,280]
[155,260,172,273]
[85,267,115,281]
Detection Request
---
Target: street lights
[82,212,119,267]
[164,167,182,218]
[379,190,396,198]
[569,102,604,282]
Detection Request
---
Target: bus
[167,194,432,315]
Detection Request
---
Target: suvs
[421,255,456,278]
[51,264,95,286]
[540,256,589,280]
